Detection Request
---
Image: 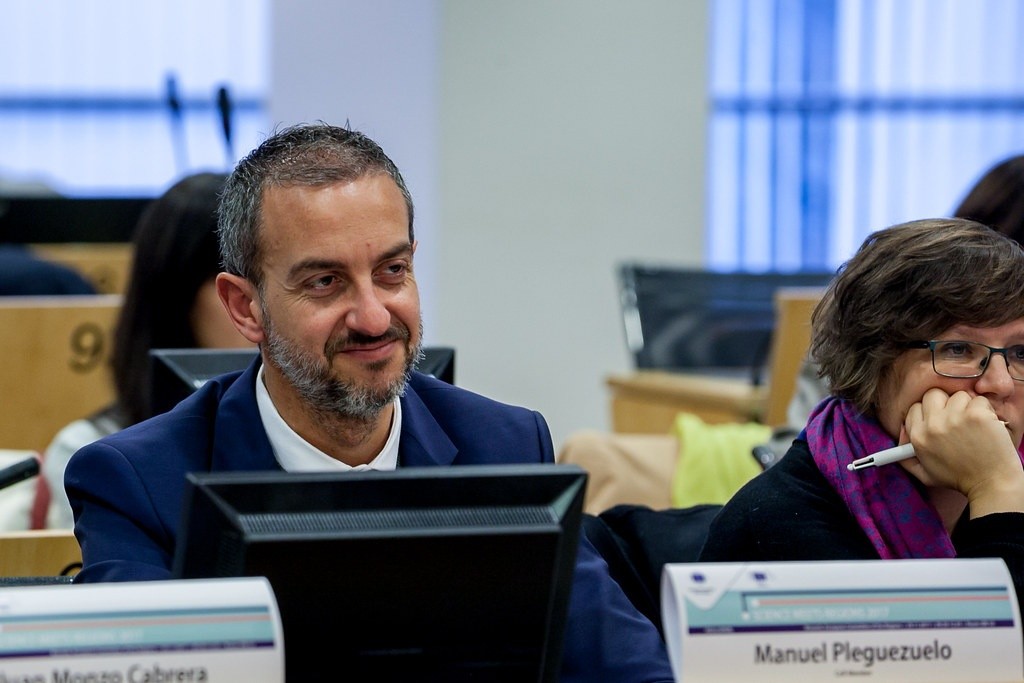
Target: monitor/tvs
[140,348,456,425]
[170,463,590,683]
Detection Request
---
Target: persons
[63,123,678,683]
[0,171,263,530]
[694,151,1024,635]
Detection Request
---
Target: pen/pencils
[845,419,1013,472]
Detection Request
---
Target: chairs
[621,266,836,379]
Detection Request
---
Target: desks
[604,369,766,435]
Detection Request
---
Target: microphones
[216,87,234,174]
[0,458,41,489]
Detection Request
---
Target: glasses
[904,338,1024,382]
